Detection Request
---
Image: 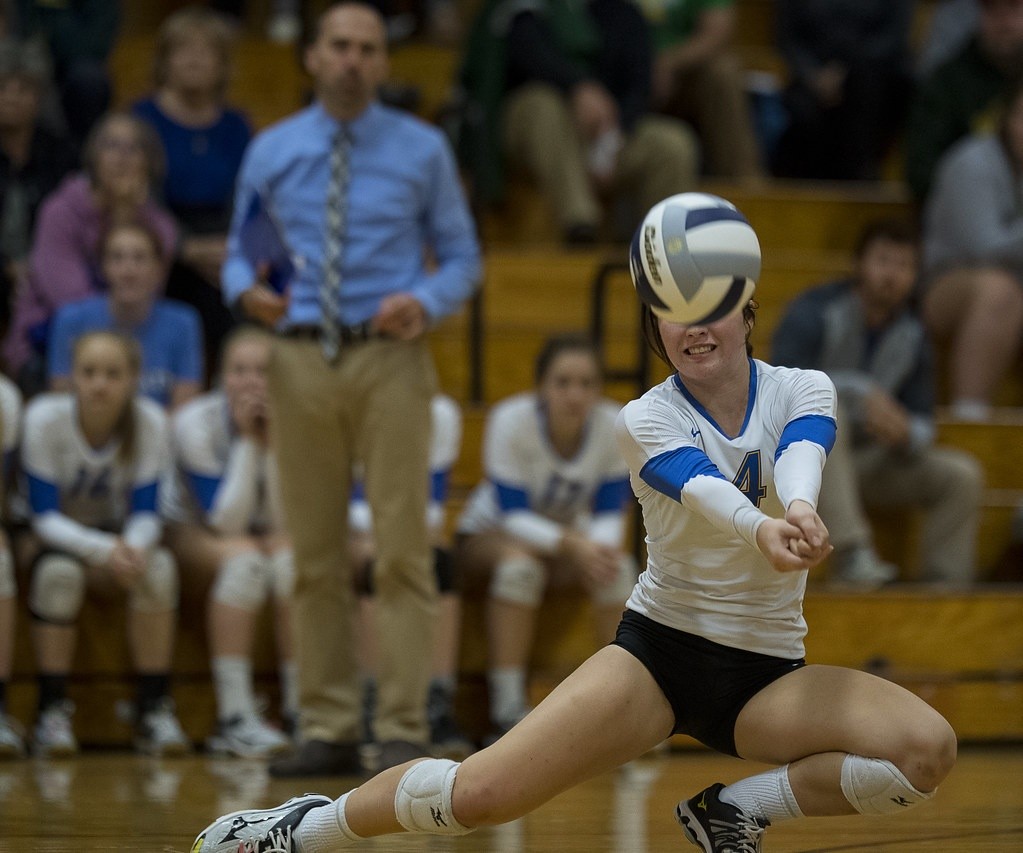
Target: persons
[456,336,641,747]
[768,217,980,593]
[221,0,485,781]
[191,194,958,852]
[0,0,1023,760]
[22,325,183,758]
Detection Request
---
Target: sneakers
[204,708,292,760]
[673,781,773,853]
[821,547,897,593]
[190,792,334,853]
[130,710,192,757]
[31,708,80,757]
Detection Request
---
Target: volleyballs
[629,191,762,329]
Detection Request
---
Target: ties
[320,126,353,365]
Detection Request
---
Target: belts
[286,323,384,345]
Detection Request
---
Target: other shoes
[1,713,28,757]
[380,739,421,771]
[273,738,364,778]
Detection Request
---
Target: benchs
[0,0,1023,750]
